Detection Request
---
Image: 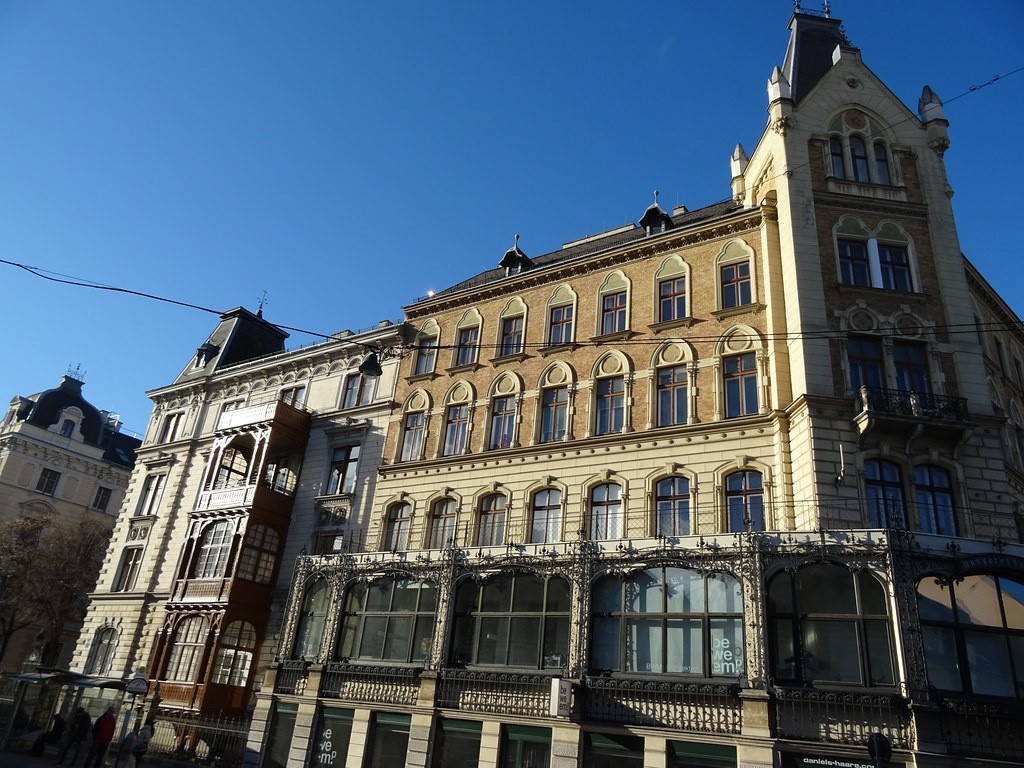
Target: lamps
[358,346,383,380]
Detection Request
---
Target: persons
[29,714,66,757]
[56,707,91,768]
[132,718,155,768]
[84,707,116,768]
